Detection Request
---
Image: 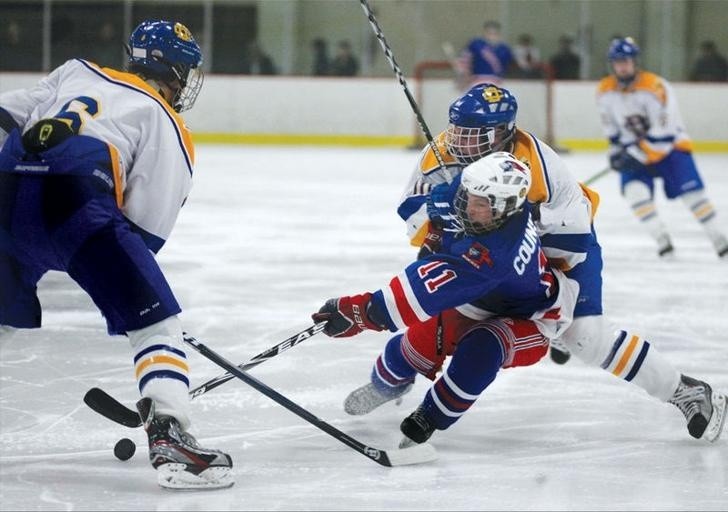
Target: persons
[238,36,358,75]
[597,36,728,256]
[398,84,713,439]
[687,41,727,82]
[0,20,234,472]
[2,21,121,73]
[451,21,582,80]
[311,150,580,446]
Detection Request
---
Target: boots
[138,398,232,468]
[669,374,711,438]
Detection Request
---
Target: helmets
[454,151,531,236]
[607,36,639,86]
[447,83,518,167]
[125,20,203,113]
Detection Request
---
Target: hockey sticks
[183,329,437,466]
[83,320,329,427]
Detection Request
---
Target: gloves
[432,172,461,228]
[312,292,384,338]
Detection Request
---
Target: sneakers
[715,237,727,256]
[658,237,671,255]
[344,382,412,415]
[400,402,435,444]
